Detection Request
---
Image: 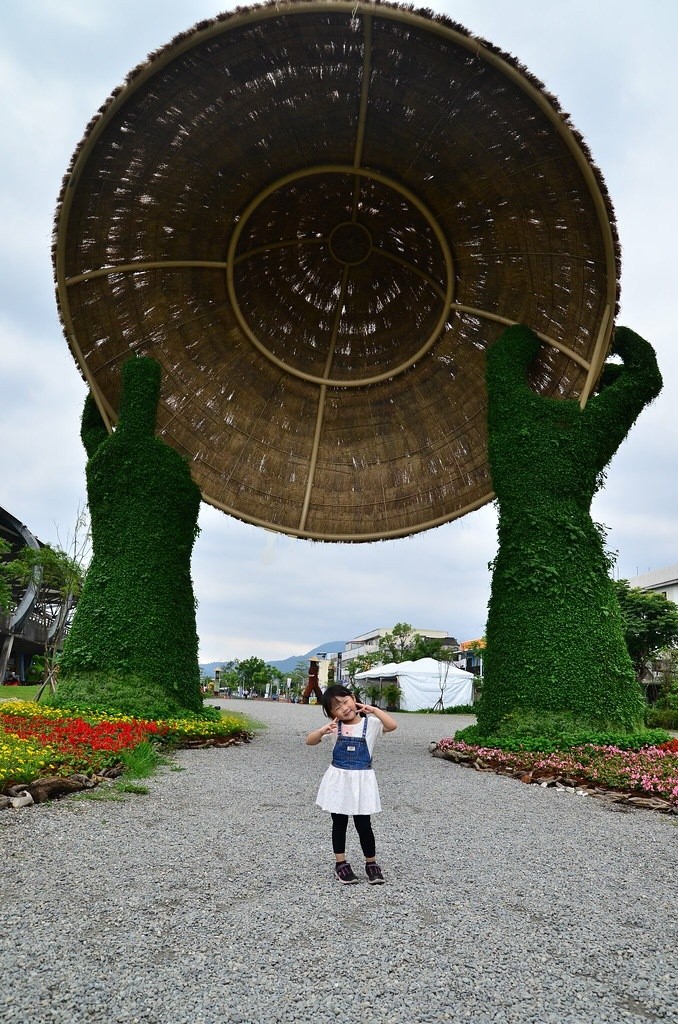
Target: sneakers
[365,863,385,883]
[334,863,359,884]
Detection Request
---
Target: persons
[306,685,397,884]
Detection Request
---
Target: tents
[353,658,474,711]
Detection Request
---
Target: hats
[51,0,621,544]
[213,667,223,671]
[308,656,321,662]
